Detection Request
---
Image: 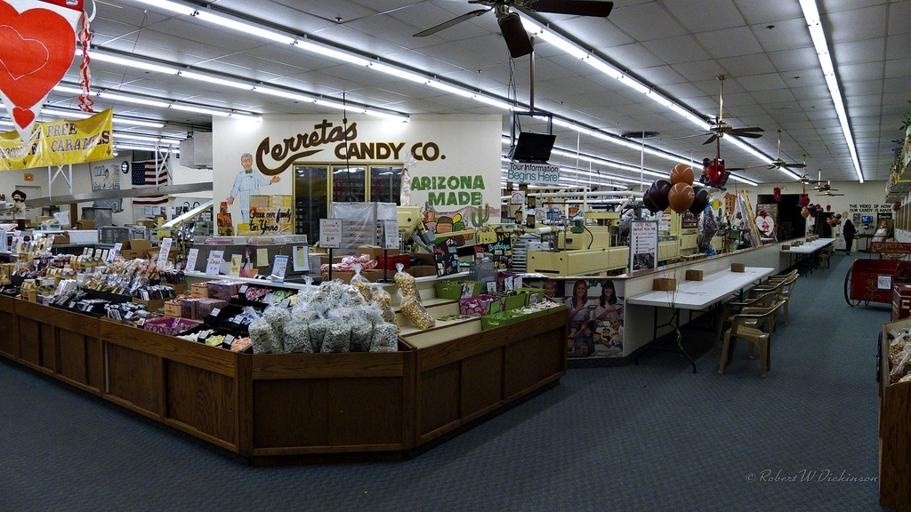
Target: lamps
[0,0,863,211]
[721,269,801,377]
[817,246,834,270]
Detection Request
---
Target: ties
[512,132,557,164]
[862,216,873,223]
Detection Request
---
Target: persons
[544,279,623,357]
[11,190,27,231]
[843,219,856,255]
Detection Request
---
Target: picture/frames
[627,265,776,373]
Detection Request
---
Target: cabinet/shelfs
[0,241,572,463]
[878,317,911,512]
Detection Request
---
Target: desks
[627,265,776,373]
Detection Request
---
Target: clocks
[121,160,128,174]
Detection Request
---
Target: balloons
[121,160,128,174]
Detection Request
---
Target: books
[319,245,437,285]
[71,218,94,230]
[120,239,149,261]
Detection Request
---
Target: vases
[881,98,910,203]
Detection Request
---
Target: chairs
[721,269,801,377]
[817,246,834,270]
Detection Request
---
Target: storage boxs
[71,218,94,230]
[319,245,437,285]
[120,239,149,261]
[780,238,838,278]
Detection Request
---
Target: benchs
[130,158,171,207]
[780,238,838,278]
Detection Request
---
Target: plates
[878,317,911,512]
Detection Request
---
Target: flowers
[881,98,910,203]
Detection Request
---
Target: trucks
[668,182,694,214]
[669,163,694,186]
[688,185,709,216]
[647,179,673,211]
[643,189,651,211]
